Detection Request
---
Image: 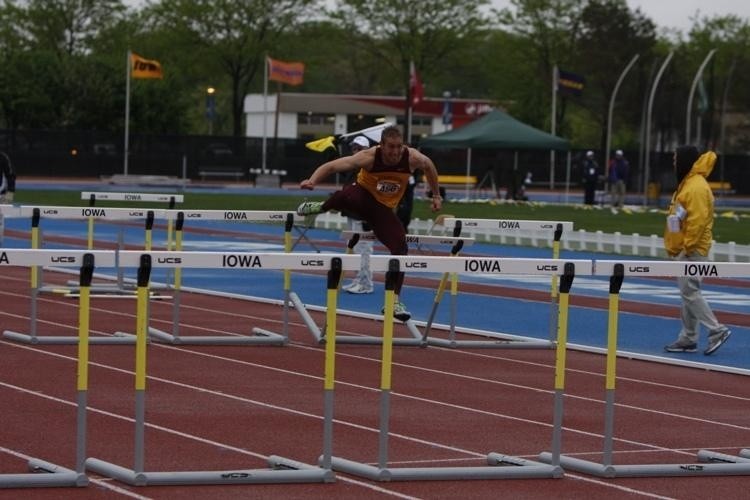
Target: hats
[615,149,624,157]
[586,151,593,156]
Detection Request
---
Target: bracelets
[432,194,441,199]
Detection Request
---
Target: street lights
[443,90,452,131]
[206,87,216,137]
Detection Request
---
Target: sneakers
[298,202,327,215]
[342,280,373,294]
[349,136,370,150]
[703,325,731,354]
[383,302,410,322]
[664,338,699,352]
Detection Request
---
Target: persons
[297,126,443,322]
[0,151,15,242]
[608,149,630,211]
[577,151,599,205]
[664,145,731,355]
[504,167,526,200]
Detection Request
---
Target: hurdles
[318,252,593,486]
[0,248,117,488]
[537,258,750,479]
[87,249,362,484]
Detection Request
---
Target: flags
[409,59,425,107]
[556,69,586,95]
[130,53,162,79]
[268,57,304,85]
